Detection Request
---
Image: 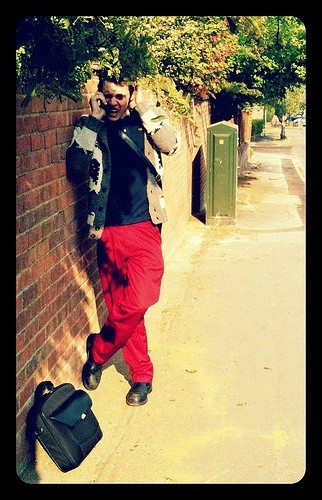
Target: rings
[96,94,98,98]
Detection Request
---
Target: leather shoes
[83,333,104,391]
[126,380,153,407]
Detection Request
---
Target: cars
[287,112,306,126]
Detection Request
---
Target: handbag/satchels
[28,380,104,472]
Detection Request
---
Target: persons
[65,69,181,405]
